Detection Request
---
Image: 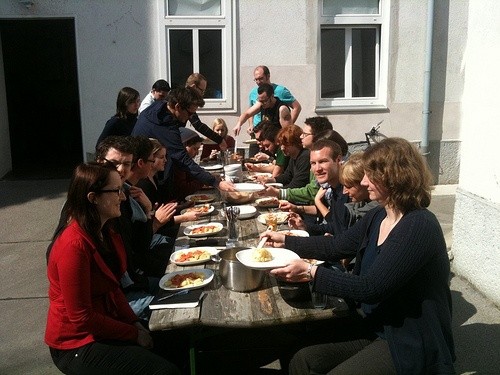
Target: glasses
[146,159,155,165]
[196,85,207,96]
[253,78,264,83]
[98,186,121,195]
[259,96,270,102]
[185,109,196,117]
[103,157,131,169]
[300,131,313,137]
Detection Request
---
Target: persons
[44,60,457,375]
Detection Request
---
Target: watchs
[307,263,315,281]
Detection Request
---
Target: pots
[210,247,266,291]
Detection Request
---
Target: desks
[148,148,349,375]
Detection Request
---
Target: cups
[227,220,240,243]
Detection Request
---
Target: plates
[181,204,215,217]
[221,205,257,218]
[244,171,275,179]
[256,195,280,206]
[281,229,310,237]
[265,181,285,188]
[184,222,223,237]
[170,246,219,267]
[185,191,215,202]
[316,259,325,266]
[258,211,294,226]
[235,247,301,270]
[242,140,257,145]
[231,183,266,191]
[159,268,215,290]
[254,162,272,167]
[199,164,223,170]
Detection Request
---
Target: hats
[149,138,162,154]
[179,127,199,144]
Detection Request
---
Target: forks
[224,207,241,218]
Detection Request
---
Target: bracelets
[300,206,305,213]
